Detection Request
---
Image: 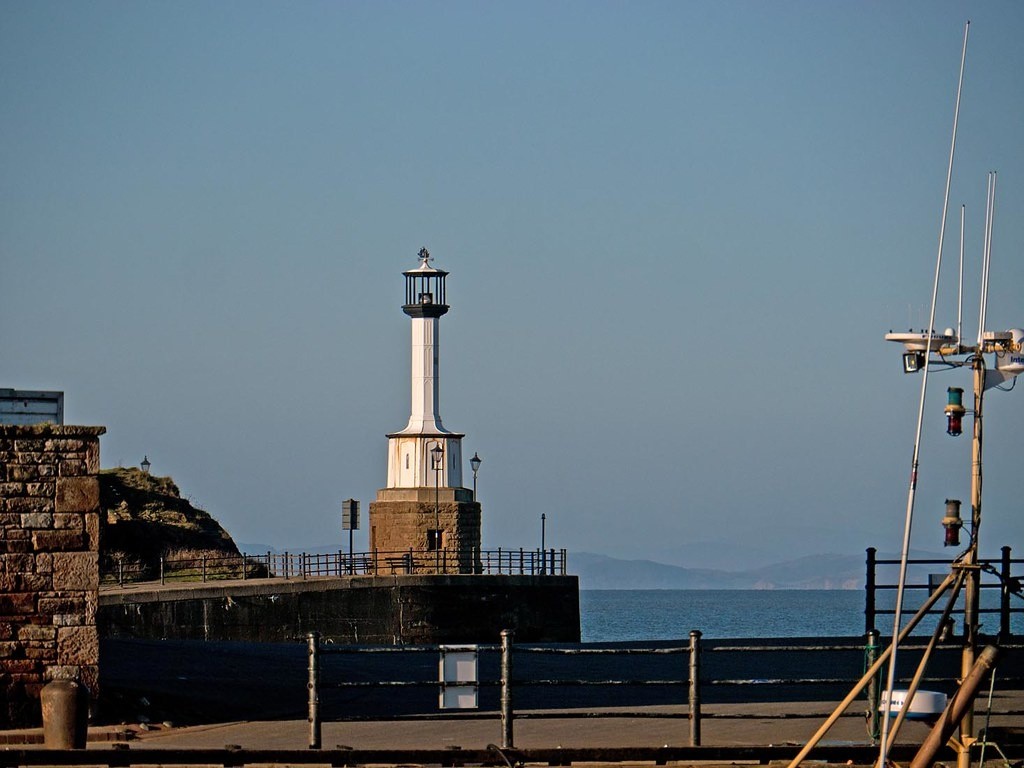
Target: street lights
[469,452,484,502]
[430,441,444,574]
